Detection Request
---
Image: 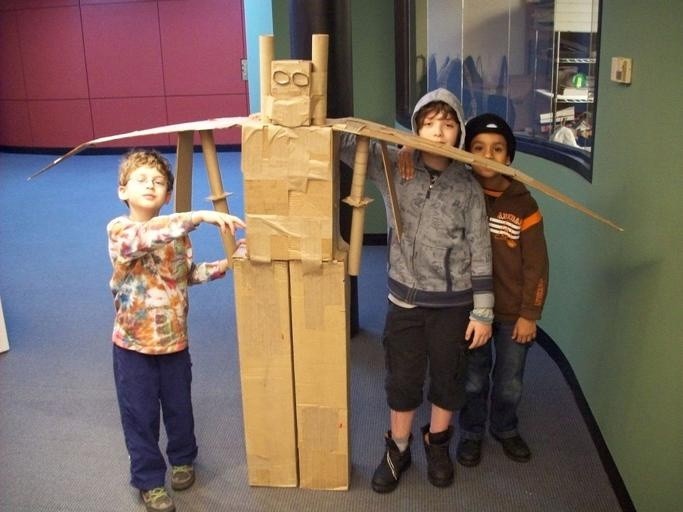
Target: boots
[423,431,454,488]
[372,431,413,493]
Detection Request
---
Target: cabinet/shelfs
[526,1,600,153]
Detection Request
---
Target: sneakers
[456,439,481,466]
[171,465,194,491]
[140,487,175,511]
[489,428,531,462]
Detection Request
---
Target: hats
[465,113,516,166]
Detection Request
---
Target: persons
[394,113,550,468]
[105,149,246,512]
[338,88,496,494]
